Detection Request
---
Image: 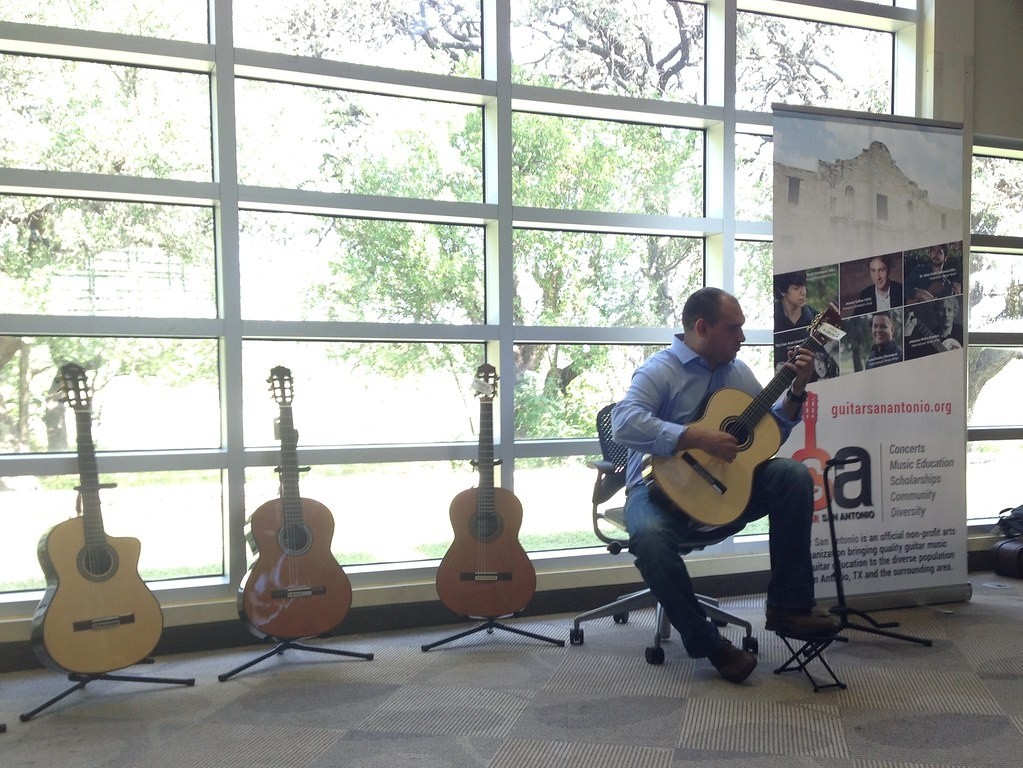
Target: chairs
[569,404,758,665]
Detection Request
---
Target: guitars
[436,364,536,618]
[30,361,166,676]
[236,364,354,643]
[650,299,844,528]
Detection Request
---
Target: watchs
[786,387,808,403]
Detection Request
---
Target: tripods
[420,457,566,652]
[803,457,932,657]
[215,466,373,681]
[19,483,195,721]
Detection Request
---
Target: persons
[865,311,903,370]
[904,297,963,361]
[853,255,902,316]
[610,287,838,682]
[774,270,820,333]
[904,243,962,305]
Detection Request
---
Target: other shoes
[764,607,840,637]
[705,635,758,683]
